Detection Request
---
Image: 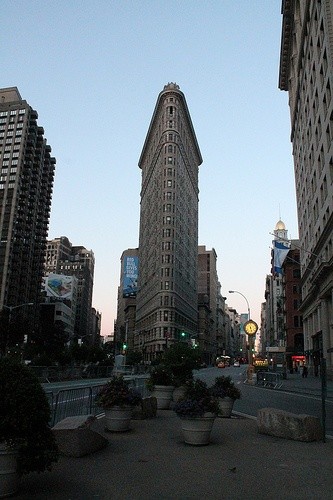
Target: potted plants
[0,356,60,500]
[144,341,192,409]
[173,355,194,408]
[207,375,242,416]
[173,377,223,445]
[86,376,144,431]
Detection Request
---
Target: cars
[218,361,225,368]
[234,362,241,367]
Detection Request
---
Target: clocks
[243,320,258,335]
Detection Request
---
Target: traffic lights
[122,341,126,351]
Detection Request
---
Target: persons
[300,365,308,378]
[294,364,298,374]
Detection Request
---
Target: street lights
[0,303,34,357]
[229,290,253,366]
